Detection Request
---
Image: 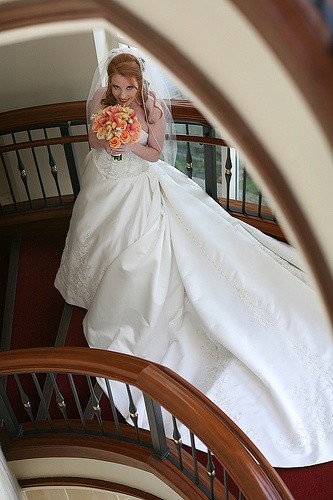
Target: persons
[53,41,167,311]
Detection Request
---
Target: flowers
[90,103,141,161]
